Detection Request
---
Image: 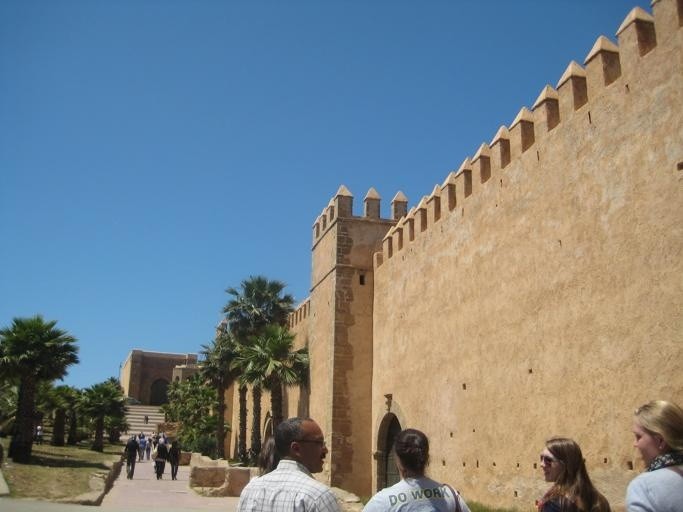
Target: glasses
[289,439,327,449]
[539,454,561,467]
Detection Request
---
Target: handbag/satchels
[152,448,158,458]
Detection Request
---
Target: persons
[36,422,43,446]
[235,416,341,512]
[143,415,146,424]
[624,399,682,512]
[250,438,275,479]
[360,428,471,511]
[124,430,180,480]
[537,437,612,512]
[145,416,149,424]
